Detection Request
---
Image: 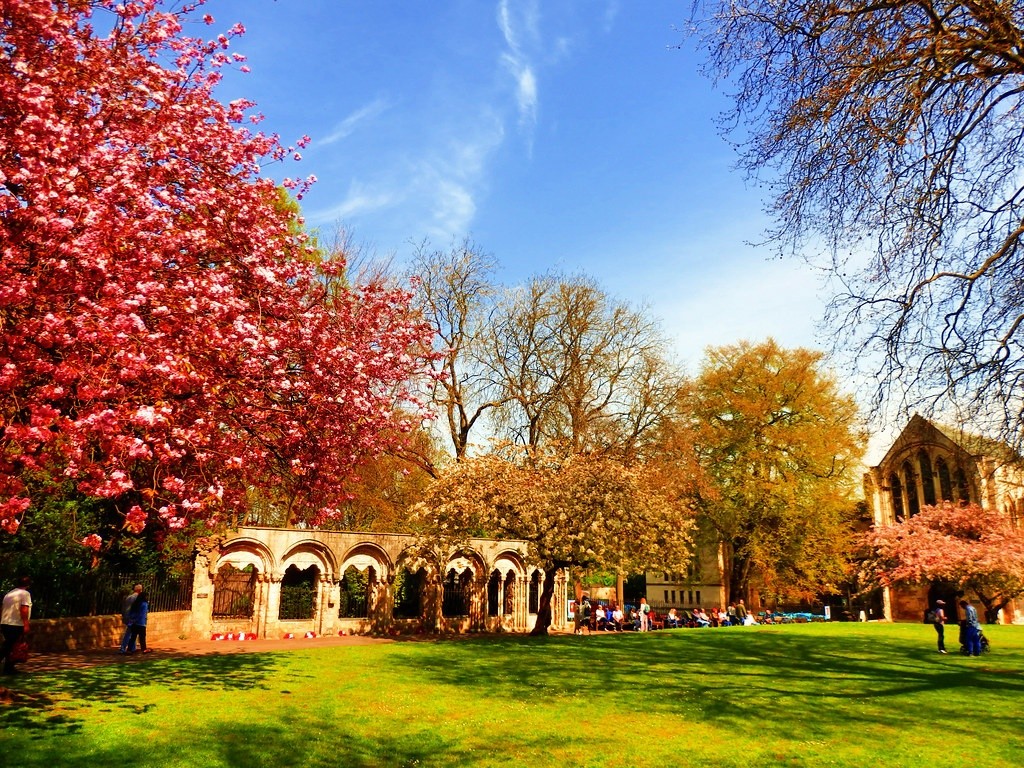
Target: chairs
[590,609,714,630]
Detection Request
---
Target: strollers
[956,619,991,653]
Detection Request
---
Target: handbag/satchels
[928,608,940,623]
[11,632,30,664]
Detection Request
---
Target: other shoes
[119,649,126,653]
[143,648,151,654]
[126,648,133,655]
[939,649,947,654]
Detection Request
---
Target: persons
[574,595,761,636]
[1,576,33,677]
[960,601,982,656]
[935,599,948,654]
[119,584,153,655]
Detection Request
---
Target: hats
[936,600,946,604]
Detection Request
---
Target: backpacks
[643,604,650,615]
